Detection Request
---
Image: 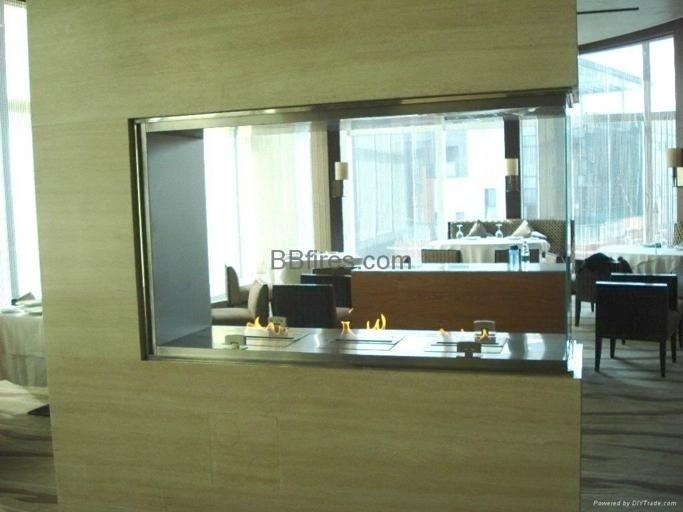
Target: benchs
[448,220,571,256]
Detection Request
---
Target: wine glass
[455,224,464,240]
[495,223,503,240]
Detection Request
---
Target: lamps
[331,161,346,198]
[503,157,520,194]
[666,147,683,186]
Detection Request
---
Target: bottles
[656,238,662,255]
[508,240,530,272]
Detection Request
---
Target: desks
[421,239,548,260]
[595,244,683,272]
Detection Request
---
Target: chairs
[420,249,460,261]
[211,280,268,326]
[594,274,678,377]
[226,266,250,305]
[574,253,631,328]
[495,248,540,263]
[271,265,358,328]
